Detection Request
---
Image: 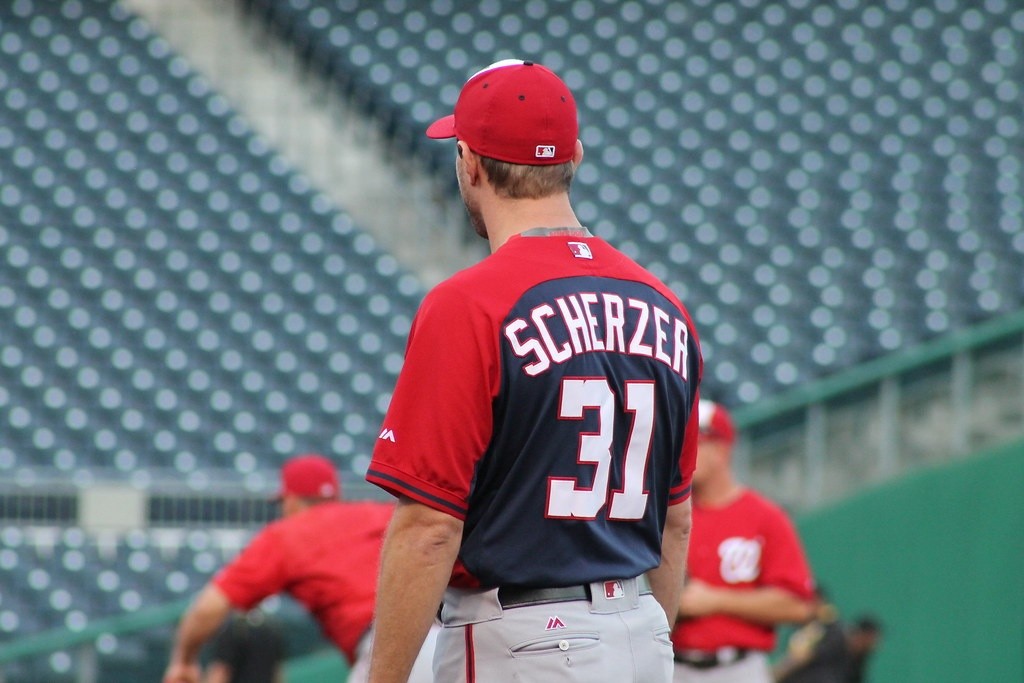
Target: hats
[268,452,342,510]
[425,59,579,166]
[698,396,740,451]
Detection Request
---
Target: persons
[366,58,704,683]
[770,585,883,683]
[164,455,440,683]
[671,399,816,683]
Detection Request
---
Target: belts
[436,572,654,625]
[674,646,752,670]
